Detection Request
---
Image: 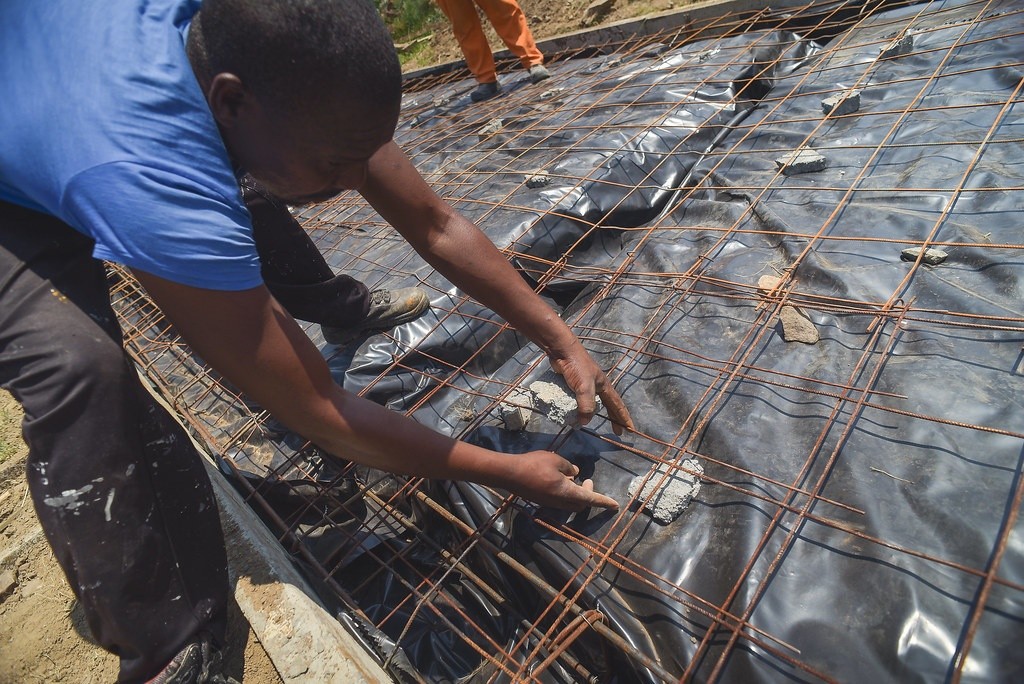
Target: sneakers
[471,80,503,103]
[143,641,242,684]
[321,287,430,345]
[528,64,550,85]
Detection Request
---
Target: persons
[435,0,550,102]
[1,0,633,684]
